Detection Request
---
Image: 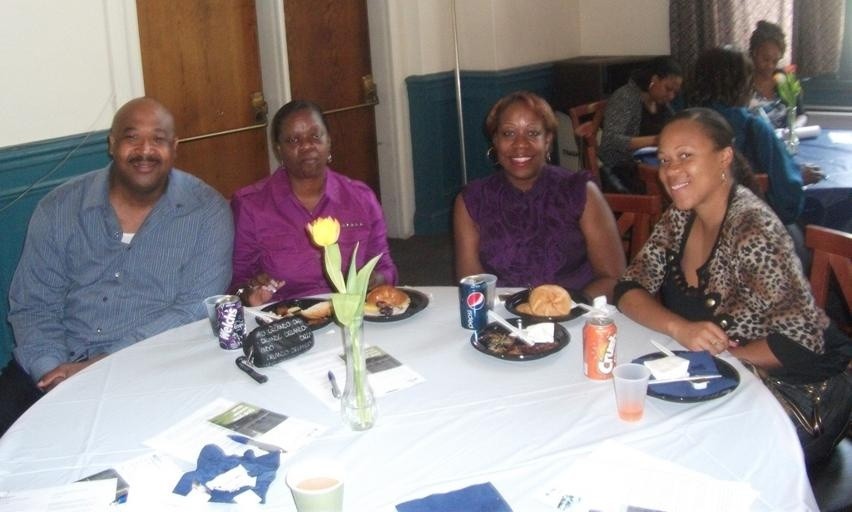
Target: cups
[612,364,649,422]
[203,295,228,338]
[283,457,344,512]
[475,275,497,311]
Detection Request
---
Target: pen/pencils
[328,371,340,398]
[226,435,287,454]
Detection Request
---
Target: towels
[634,349,736,397]
[397,482,512,512]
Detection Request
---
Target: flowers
[306,214,384,427]
[772,63,802,134]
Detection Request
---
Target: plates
[471,318,570,361]
[505,287,592,322]
[255,298,332,329]
[363,288,429,322]
[633,351,740,403]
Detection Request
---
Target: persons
[742,21,810,129]
[229,98,399,307]
[693,47,824,224]
[454,92,627,306]
[595,57,684,193]
[613,108,852,466]
[1,97,235,433]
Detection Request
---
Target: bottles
[460,275,488,329]
[583,315,617,380]
[215,295,246,350]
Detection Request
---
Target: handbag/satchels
[235,316,315,385]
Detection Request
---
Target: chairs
[603,193,661,267]
[803,224,852,341]
[568,99,607,156]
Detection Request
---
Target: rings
[710,335,720,344]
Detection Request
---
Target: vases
[339,321,377,432]
[782,108,794,153]
